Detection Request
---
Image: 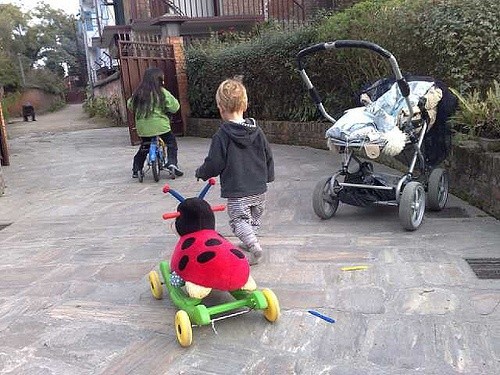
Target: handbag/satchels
[325,93,395,142]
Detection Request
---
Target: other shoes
[246,244,262,266]
[239,242,248,250]
[133,171,144,178]
[167,164,183,176]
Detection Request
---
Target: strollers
[296,40,458,231]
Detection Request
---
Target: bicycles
[137,135,175,182]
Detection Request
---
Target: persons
[22,102,37,122]
[127,67,184,178]
[195,75,275,266]
